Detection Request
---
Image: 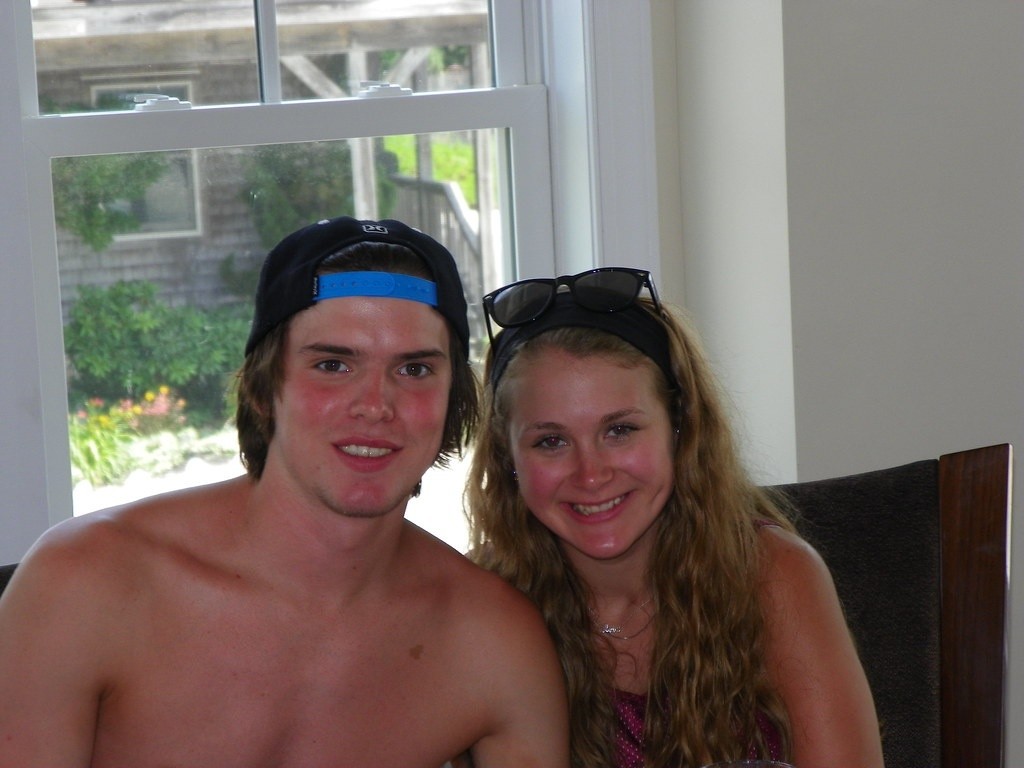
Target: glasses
[482,266,665,357]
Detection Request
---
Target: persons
[0,219,570,768]
[445,292,883,768]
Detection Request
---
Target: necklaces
[588,597,655,634]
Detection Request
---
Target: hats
[244,216,470,364]
